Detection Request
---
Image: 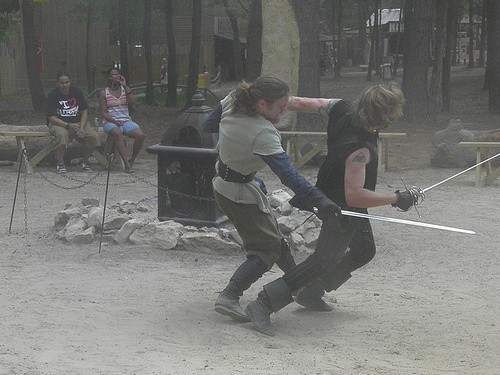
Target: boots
[245,276,294,336]
[295,263,352,312]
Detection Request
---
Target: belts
[218,155,257,184]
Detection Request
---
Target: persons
[202,75,341,321]
[45,72,96,175]
[99,68,144,173]
[246,86,413,336]
[160,58,168,91]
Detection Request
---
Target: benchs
[279,131,406,176]
[458,142,500,186]
[0,131,129,175]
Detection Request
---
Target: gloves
[318,199,340,220]
[392,189,413,210]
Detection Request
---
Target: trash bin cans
[146,89,231,227]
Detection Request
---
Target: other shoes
[78,161,94,174]
[125,160,135,172]
[56,164,66,173]
[214,291,247,321]
